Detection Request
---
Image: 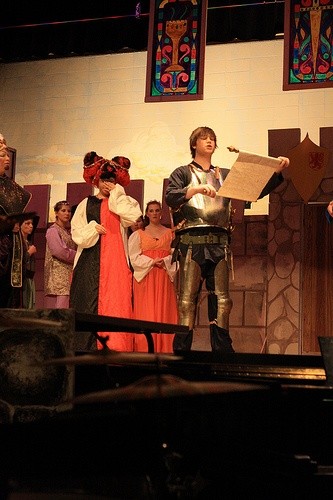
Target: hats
[82,151,130,188]
[0,134,16,152]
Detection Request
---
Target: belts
[180,233,228,244]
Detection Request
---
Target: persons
[0,132,180,354]
[165,126,289,362]
[326,200,333,225]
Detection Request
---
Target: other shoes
[176,350,191,360]
[209,352,238,363]
